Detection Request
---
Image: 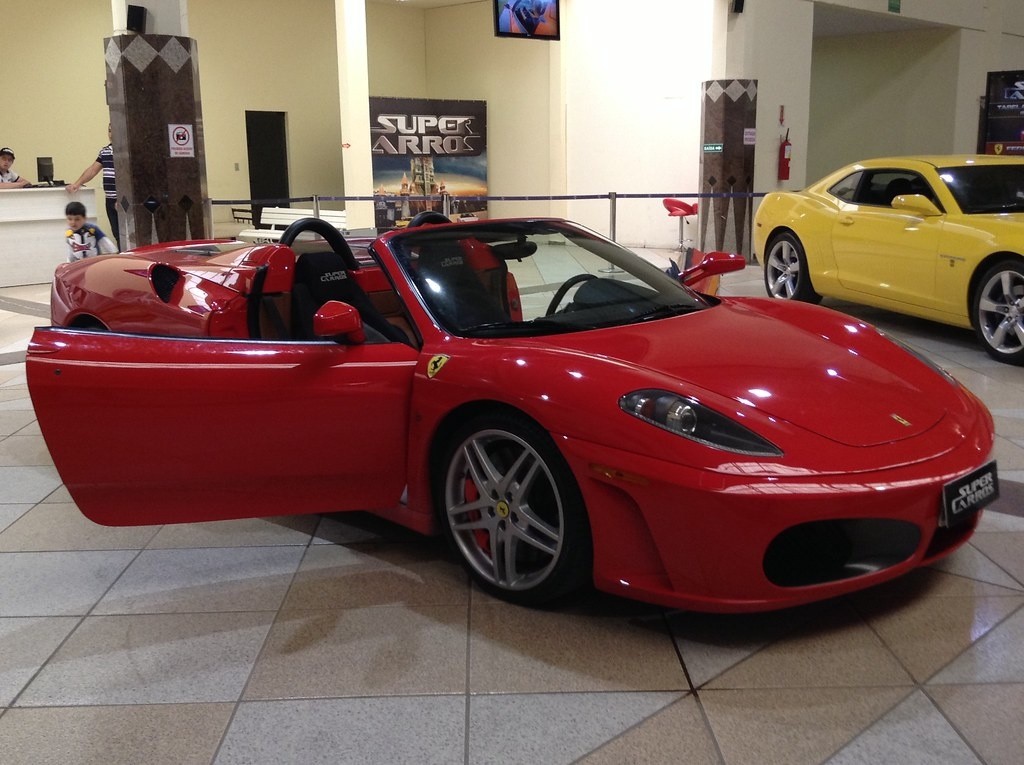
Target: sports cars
[505,0,557,36]
[25,210,1001,615]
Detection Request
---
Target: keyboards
[32,185,38,187]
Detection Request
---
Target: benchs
[238,206,347,245]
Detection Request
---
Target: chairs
[406,211,495,344]
[291,250,414,347]
[883,178,914,206]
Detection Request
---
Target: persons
[65,122,121,252]
[0,147,30,189]
[65,201,119,263]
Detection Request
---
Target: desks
[0,185,97,288]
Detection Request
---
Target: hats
[0,148,14,158]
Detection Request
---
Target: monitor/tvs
[492,0,560,41]
[37,157,56,187]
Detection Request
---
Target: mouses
[23,184,33,188]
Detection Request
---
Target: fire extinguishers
[778,128,792,181]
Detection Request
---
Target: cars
[751,155,1024,365]
[396,217,413,227]
[457,213,479,222]
